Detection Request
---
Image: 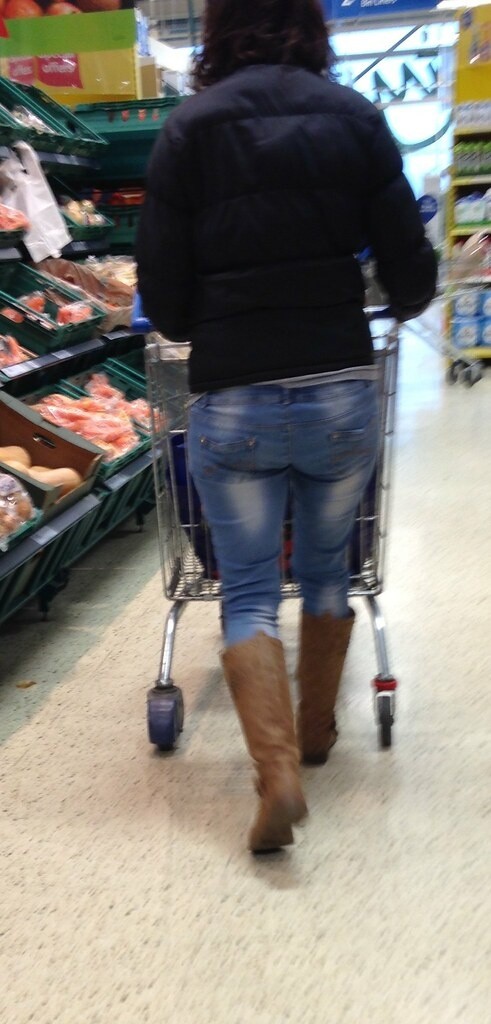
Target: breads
[0,446,83,502]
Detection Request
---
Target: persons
[132,0,441,855]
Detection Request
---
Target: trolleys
[144,296,406,753]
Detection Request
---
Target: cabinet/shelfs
[442,125,490,360]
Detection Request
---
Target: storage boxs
[0,8,193,629]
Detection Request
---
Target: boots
[219,630,308,851]
[296,605,356,765]
